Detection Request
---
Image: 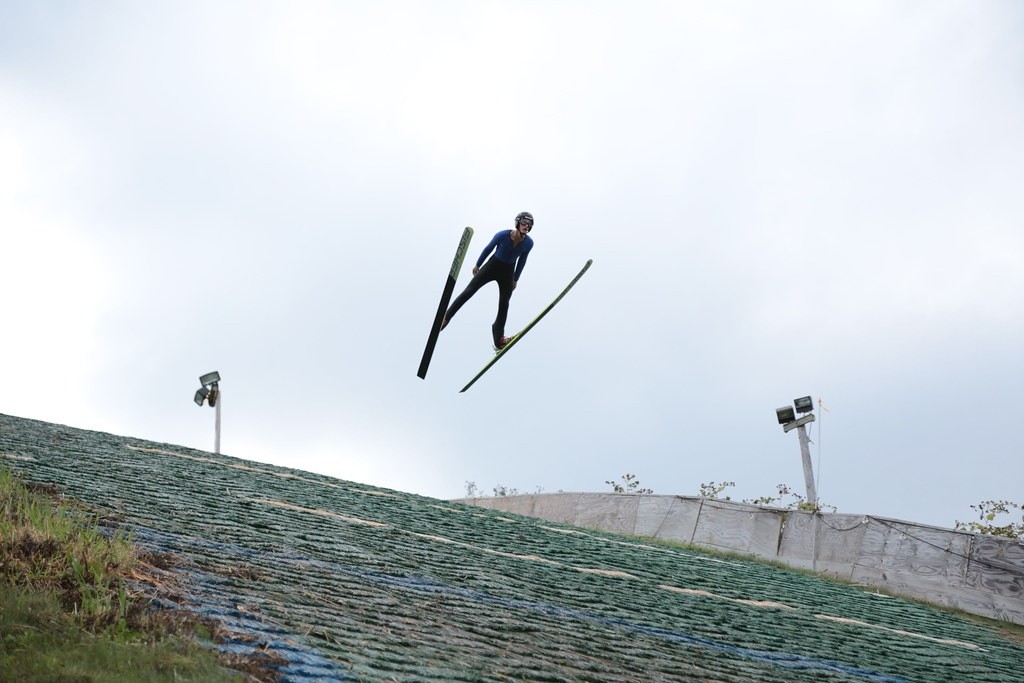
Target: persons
[440,212,533,352]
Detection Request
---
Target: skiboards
[416,226,594,393]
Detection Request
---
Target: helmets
[515,212,533,232]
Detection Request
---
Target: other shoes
[441,319,448,332]
[494,336,515,351]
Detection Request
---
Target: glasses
[520,219,532,228]
